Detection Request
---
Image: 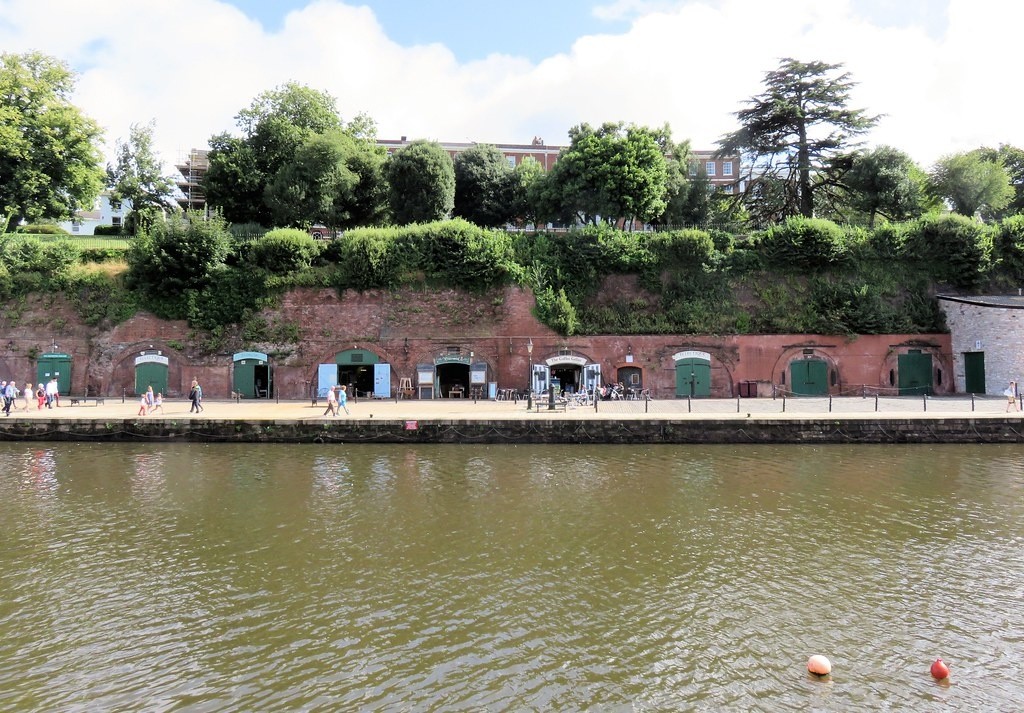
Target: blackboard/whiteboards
[471,371,486,384]
[419,386,434,399]
[488,381,497,400]
[417,371,433,384]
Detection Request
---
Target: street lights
[526,338,534,410]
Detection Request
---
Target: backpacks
[38,388,44,397]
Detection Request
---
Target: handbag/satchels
[1004,390,1014,397]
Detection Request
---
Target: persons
[23,383,33,412]
[151,393,164,415]
[336,386,350,415]
[190,380,204,413]
[596,382,650,401]
[35,377,60,409]
[575,385,589,405]
[323,386,338,417]
[138,386,154,416]
[0,380,19,417]
[1006,381,1019,412]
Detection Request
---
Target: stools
[497,394,506,401]
[523,394,528,400]
[513,394,521,400]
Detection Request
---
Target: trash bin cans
[749,381,757,397]
[740,382,748,397]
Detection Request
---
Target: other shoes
[333,413,336,417]
[7,414,9,416]
[322,414,328,418]
[49,407,52,409]
[138,414,141,416]
[348,413,352,415]
[143,414,146,416]
[336,412,340,416]
[45,403,47,407]
[190,410,192,412]
[196,411,199,413]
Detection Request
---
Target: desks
[449,391,463,398]
[418,384,435,400]
[499,389,517,400]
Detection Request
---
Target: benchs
[535,403,566,413]
[312,388,329,407]
[65,396,105,407]
